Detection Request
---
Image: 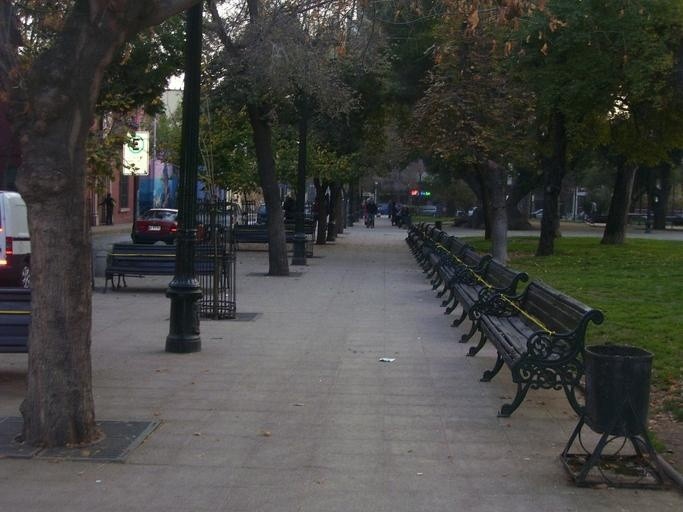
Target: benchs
[406,222,603,420]
[628,208,683,230]
[103,215,318,292]
[0,288,31,353]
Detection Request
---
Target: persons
[590,200,599,223]
[283,191,330,224]
[95,192,116,225]
[363,196,410,228]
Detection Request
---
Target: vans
[0,189,35,290]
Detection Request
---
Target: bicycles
[365,212,374,230]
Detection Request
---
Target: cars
[256,203,268,226]
[131,206,205,246]
[283,215,317,234]
[196,203,246,232]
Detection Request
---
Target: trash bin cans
[435,221,442,230]
[560,342,674,490]
